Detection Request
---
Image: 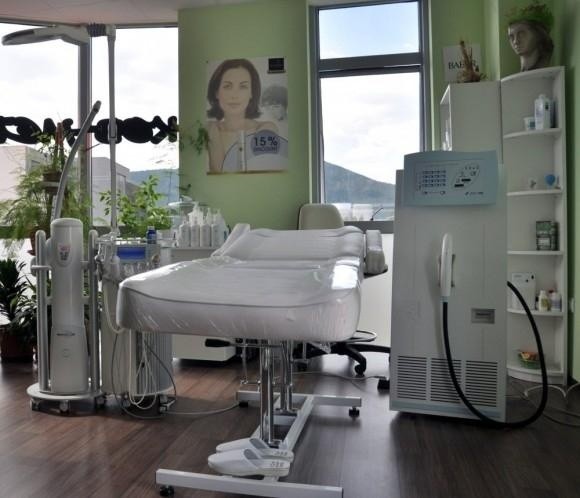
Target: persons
[507,19,554,71]
[204,59,289,172]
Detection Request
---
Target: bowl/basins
[518,353,541,369]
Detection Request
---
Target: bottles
[538,290,562,312]
[146,226,157,244]
[179,221,223,248]
[534,94,551,131]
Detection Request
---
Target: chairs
[288,203,390,375]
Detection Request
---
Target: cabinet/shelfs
[167,246,237,367]
[437,84,500,154]
[500,67,569,389]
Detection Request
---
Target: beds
[116,223,385,498]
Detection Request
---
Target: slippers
[207,437,294,478]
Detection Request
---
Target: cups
[523,117,534,131]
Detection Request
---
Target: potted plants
[0,129,93,364]
